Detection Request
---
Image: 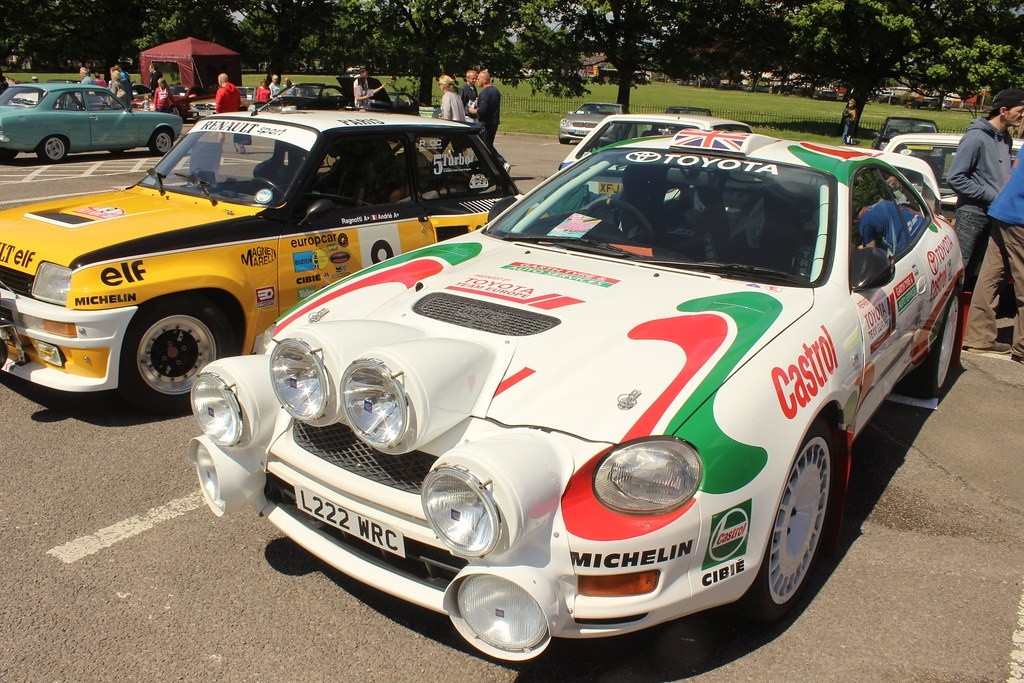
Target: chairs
[588,106,599,114]
[396,150,431,175]
[641,130,663,137]
[245,160,286,194]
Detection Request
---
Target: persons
[0,70,14,96]
[79,61,301,154]
[842,99,856,146]
[964,142,1024,363]
[946,88,1024,292]
[354,69,386,112]
[437,71,510,174]
[856,177,924,259]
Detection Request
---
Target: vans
[880,91,895,97]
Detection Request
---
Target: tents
[139,36,243,88]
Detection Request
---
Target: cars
[559,114,755,195]
[558,102,637,144]
[675,79,771,93]
[882,134,1023,225]
[188,86,265,118]
[0,82,185,164]
[773,84,838,100]
[919,98,952,109]
[873,116,945,177]
[258,75,420,116]
[189,129,968,662]
[131,84,198,121]
[0,103,544,392]
[651,106,712,134]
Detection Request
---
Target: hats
[31,76,39,80]
[990,88,1024,112]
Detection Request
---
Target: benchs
[887,129,918,134]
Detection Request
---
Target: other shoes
[968,340,1012,355]
[1011,355,1024,364]
[503,163,511,173]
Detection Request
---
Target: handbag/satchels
[107,83,127,106]
